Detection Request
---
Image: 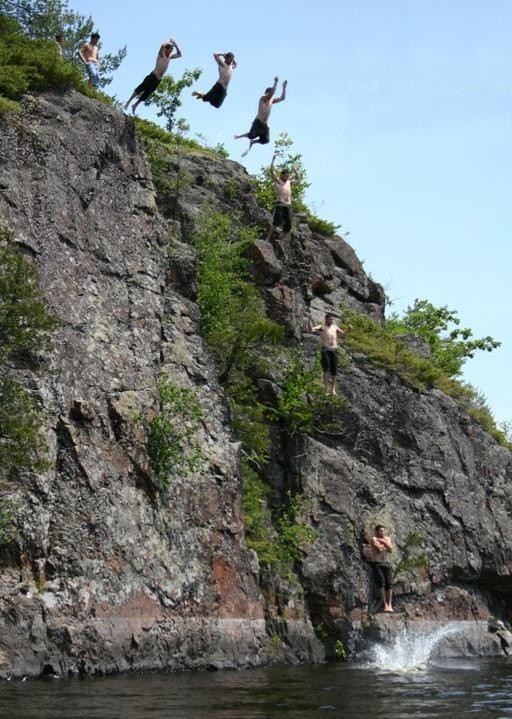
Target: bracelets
[272,156,275,159]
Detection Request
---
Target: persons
[266,150,301,241]
[307,313,352,395]
[55,34,64,55]
[78,33,100,88]
[124,38,182,115]
[373,524,395,613]
[234,76,287,157]
[192,51,238,108]
